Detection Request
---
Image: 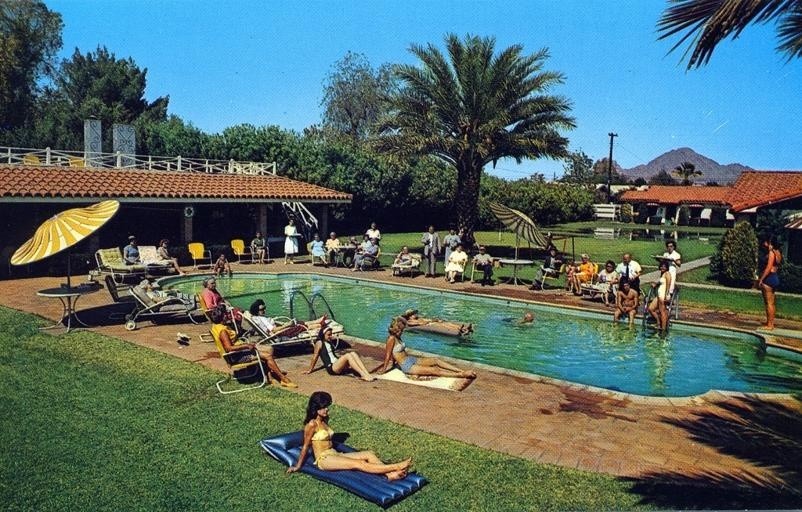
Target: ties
[626,263,629,279]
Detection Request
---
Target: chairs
[69,157,84,167]
[540,263,646,303]
[445,255,468,282]
[392,253,422,278]
[471,257,493,283]
[24,153,40,165]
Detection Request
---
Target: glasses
[260,306,266,311]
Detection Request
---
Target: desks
[37,288,99,332]
[499,259,533,286]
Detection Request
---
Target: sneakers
[176,332,191,346]
[283,260,294,264]
[280,380,297,389]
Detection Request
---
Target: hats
[547,246,559,252]
[402,308,418,316]
[761,235,784,247]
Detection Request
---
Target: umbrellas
[5,197,121,290]
[484,200,547,259]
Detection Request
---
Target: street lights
[606,133,619,204]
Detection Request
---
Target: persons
[208,304,298,388]
[402,307,474,336]
[213,254,231,278]
[472,245,495,287]
[650,240,682,303]
[756,231,782,330]
[201,277,231,310]
[572,252,594,299]
[613,281,639,328]
[308,221,381,273]
[565,258,578,293]
[443,222,462,270]
[615,252,643,297]
[536,230,557,252]
[139,278,201,311]
[421,223,442,278]
[648,261,672,333]
[595,260,621,306]
[396,245,420,272]
[375,315,476,380]
[154,239,187,275]
[301,326,377,382]
[528,247,567,290]
[283,219,305,265]
[122,234,155,279]
[518,312,533,324]
[250,299,329,338]
[251,230,268,265]
[286,391,412,482]
[444,243,469,282]
